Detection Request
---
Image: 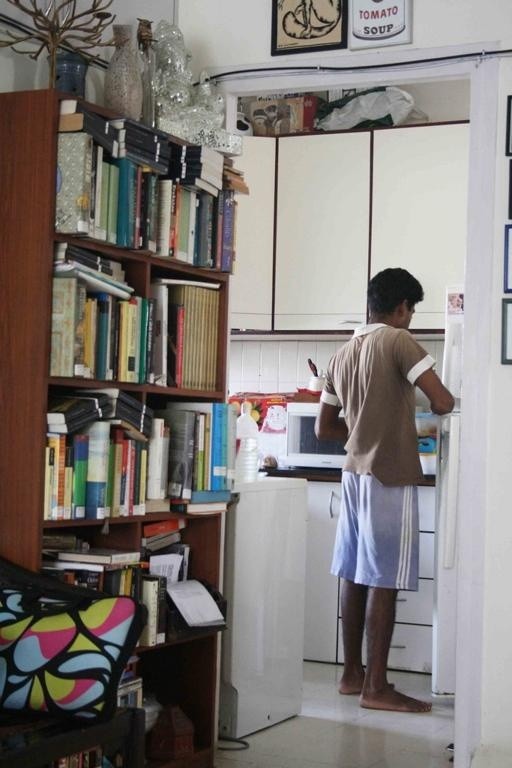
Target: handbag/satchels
[0,552,149,727]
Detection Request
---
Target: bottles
[237,405,259,482]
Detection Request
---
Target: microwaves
[286,402,347,468]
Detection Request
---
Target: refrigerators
[431,321,463,698]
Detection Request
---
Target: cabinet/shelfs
[372,123,470,337]
[223,136,276,332]
[345,486,441,673]
[5,94,234,767]
[271,134,374,336]
[307,483,341,665]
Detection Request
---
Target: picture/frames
[347,0,414,51]
[272,0,348,56]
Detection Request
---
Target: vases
[129,19,160,127]
[105,23,143,118]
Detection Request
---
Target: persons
[314,268,455,714]
[235,402,260,488]
[251,104,290,136]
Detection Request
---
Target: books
[45,521,227,648]
[42,387,239,521]
[48,744,130,768]
[48,243,222,396]
[53,97,251,275]
[118,676,162,734]
